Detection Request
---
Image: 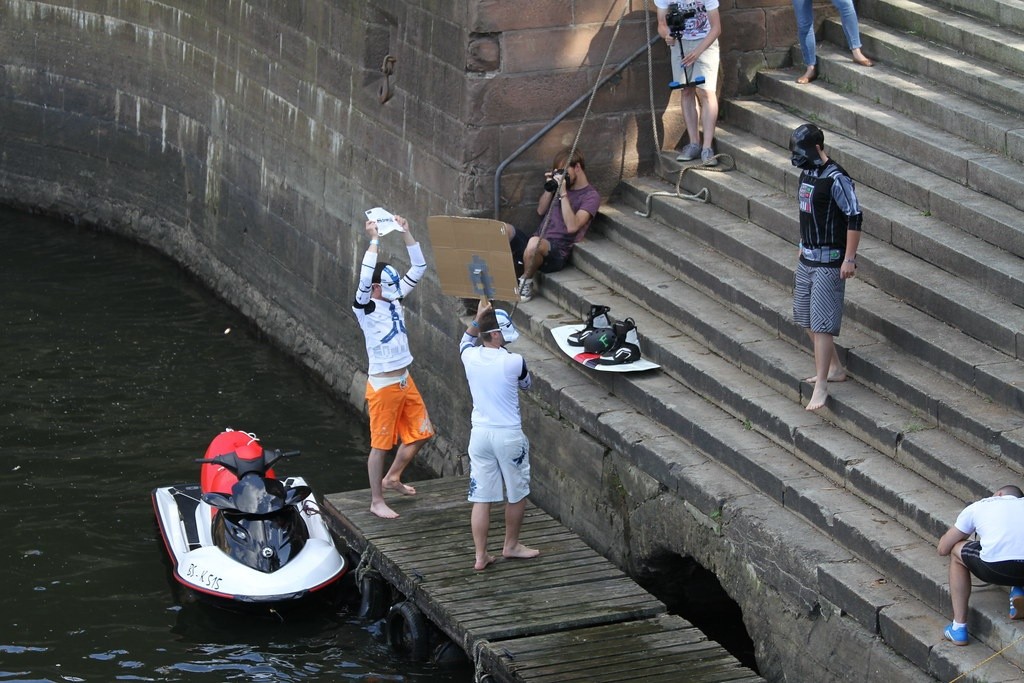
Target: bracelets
[369,239,380,245]
[471,320,481,329]
[844,258,857,264]
[558,192,568,200]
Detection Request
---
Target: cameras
[543,168,570,193]
[665,4,697,32]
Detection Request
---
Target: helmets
[584,328,617,355]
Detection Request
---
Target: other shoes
[853,55,873,66]
[798,73,817,84]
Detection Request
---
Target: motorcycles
[150,429,348,619]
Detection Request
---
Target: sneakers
[944,623,968,646]
[1009,587,1024,619]
[676,143,702,160]
[701,148,718,166]
[600,317,641,365]
[567,305,611,346]
[518,274,535,303]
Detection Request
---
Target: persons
[458,299,541,570]
[500,146,601,304]
[788,123,863,412]
[936,484,1024,646]
[350,214,435,518]
[792,0,874,84]
[652,0,723,166]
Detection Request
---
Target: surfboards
[550,305,662,373]
